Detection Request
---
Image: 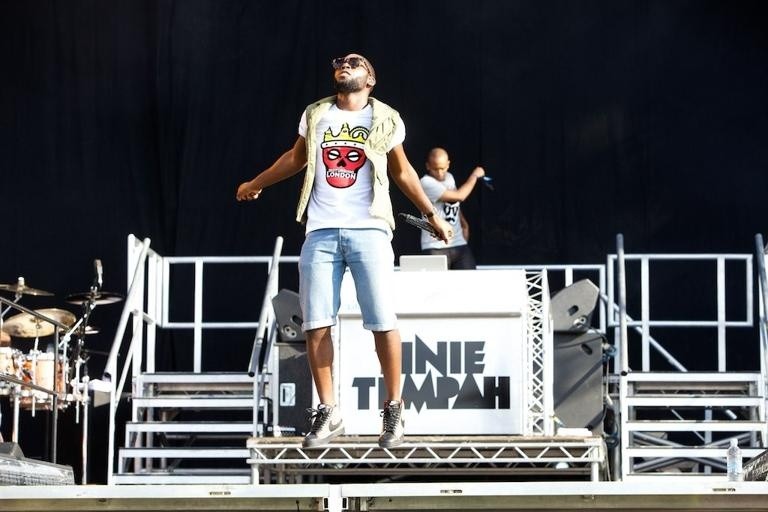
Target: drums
[0,347,25,395]
[19,353,67,410]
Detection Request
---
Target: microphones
[400,210,452,243]
[93,260,104,289]
[478,174,499,194]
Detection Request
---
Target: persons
[421,147,486,269]
[236,53,455,448]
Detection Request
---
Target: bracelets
[424,207,438,220]
[470,173,480,179]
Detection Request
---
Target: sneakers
[302,403,345,447]
[379,399,405,447]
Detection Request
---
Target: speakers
[533,333,611,435]
[549,278,600,332]
[272,344,313,437]
[271,290,309,343]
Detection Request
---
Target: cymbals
[2,308,76,337]
[62,293,123,305]
[0,285,53,296]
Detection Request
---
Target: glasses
[333,58,370,75]
